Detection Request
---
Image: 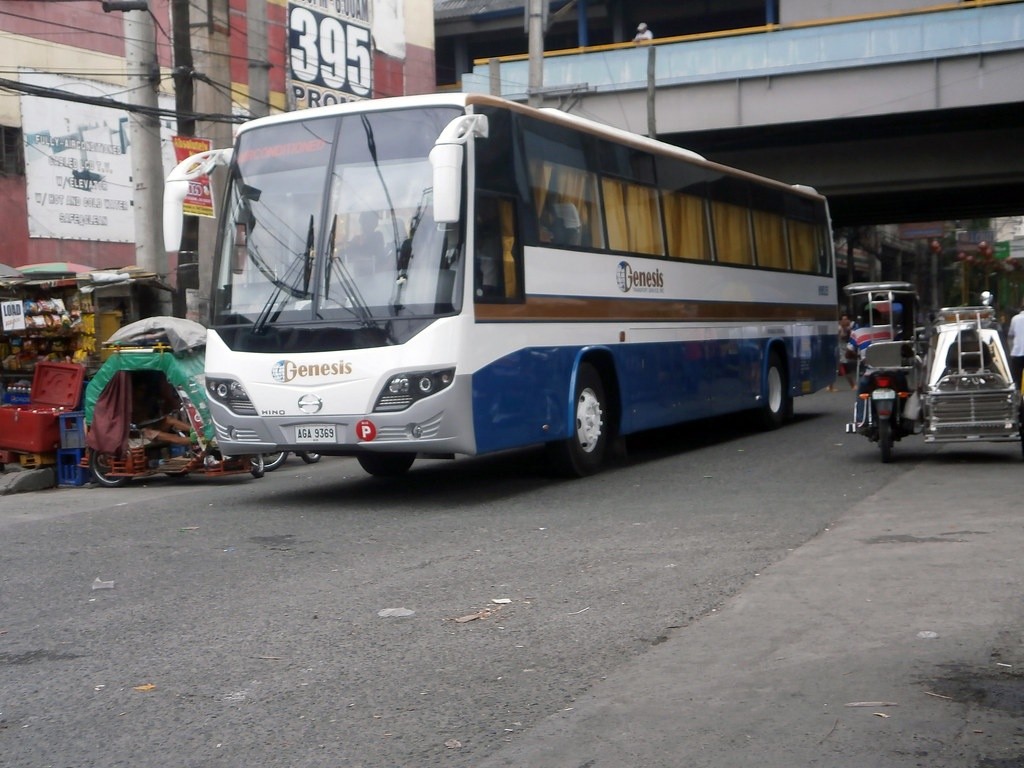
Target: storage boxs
[56,411,90,487]
[0,362,86,453]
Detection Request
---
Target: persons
[826,314,860,392]
[346,211,383,276]
[634,23,652,48]
[130,413,191,446]
[1008,307,1024,389]
[845,308,897,360]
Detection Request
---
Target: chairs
[554,202,583,246]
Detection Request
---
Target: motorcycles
[844,282,1024,464]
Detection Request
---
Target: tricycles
[87,342,265,488]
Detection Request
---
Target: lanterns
[957,241,1022,276]
[931,241,941,254]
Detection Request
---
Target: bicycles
[241,449,320,472]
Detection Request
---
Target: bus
[196,91,840,478]
